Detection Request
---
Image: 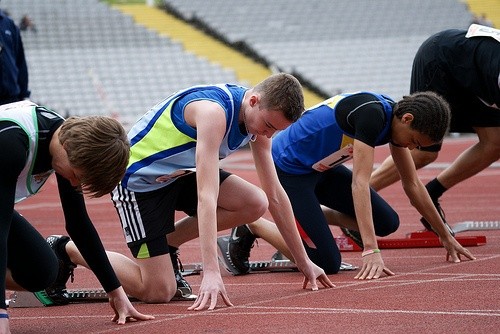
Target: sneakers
[44,234,77,306]
[418,201,454,239]
[341,226,363,249]
[225,223,256,275]
[167,247,194,294]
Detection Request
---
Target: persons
[0,99,155,334]
[32,72,336,311]
[227,90,476,280]
[341,24,500,252]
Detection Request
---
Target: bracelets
[362,248,381,257]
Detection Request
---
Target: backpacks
[0,8,32,104]
